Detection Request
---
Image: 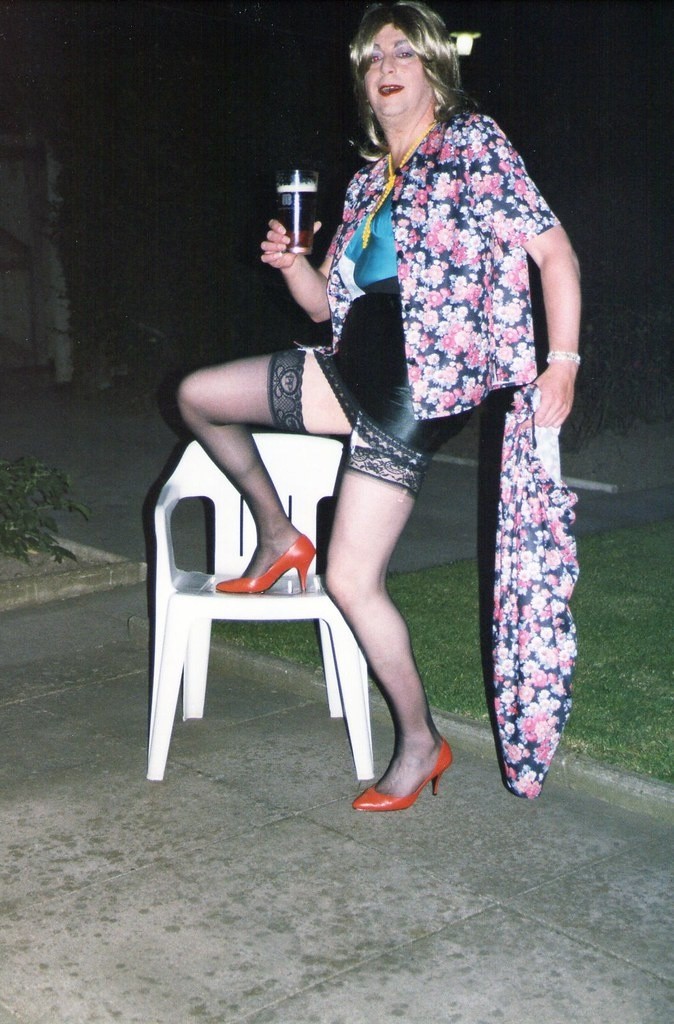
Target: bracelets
[547,351,581,364]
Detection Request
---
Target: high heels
[352,736,453,811]
[215,533,316,593]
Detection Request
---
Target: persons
[179,1,583,813]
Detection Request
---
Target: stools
[145,430,375,782]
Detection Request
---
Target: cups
[276,169,319,254]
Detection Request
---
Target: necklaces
[362,120,437,249]
